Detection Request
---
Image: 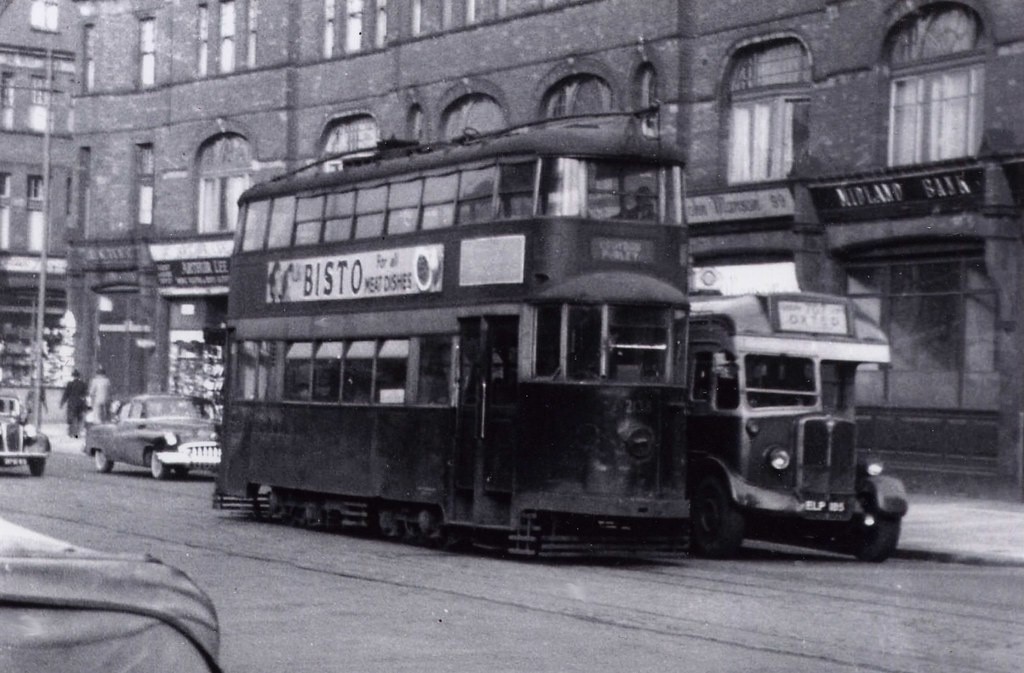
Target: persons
[26,388,49,415]
[314,368,339,401]
[629,186,656,220]
[288,382,309,401]
[91,368,110,423]
[60,371,88,438]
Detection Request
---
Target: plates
[413,251,432,291]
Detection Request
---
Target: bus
[213,104,694,558]
[683,288,910,567]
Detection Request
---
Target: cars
[83,392,224,483]
[0,387,52,478]
